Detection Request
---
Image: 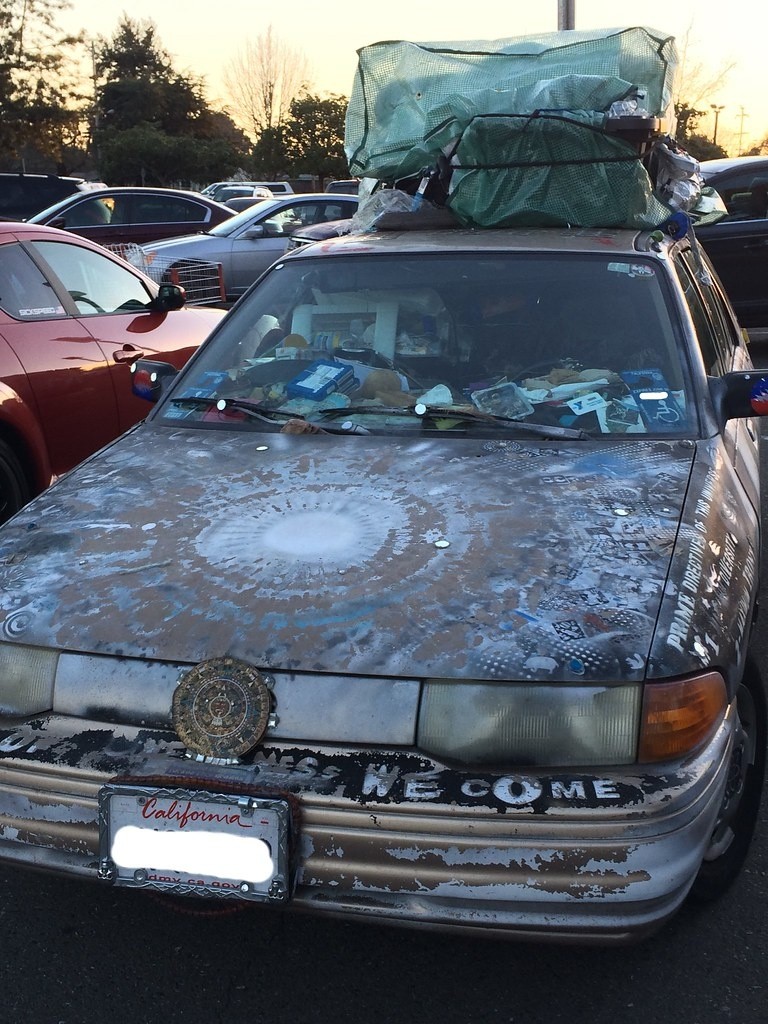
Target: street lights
[711,103,724,145]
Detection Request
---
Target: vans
[201,182,296,206]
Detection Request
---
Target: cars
[0,156,768,952]
